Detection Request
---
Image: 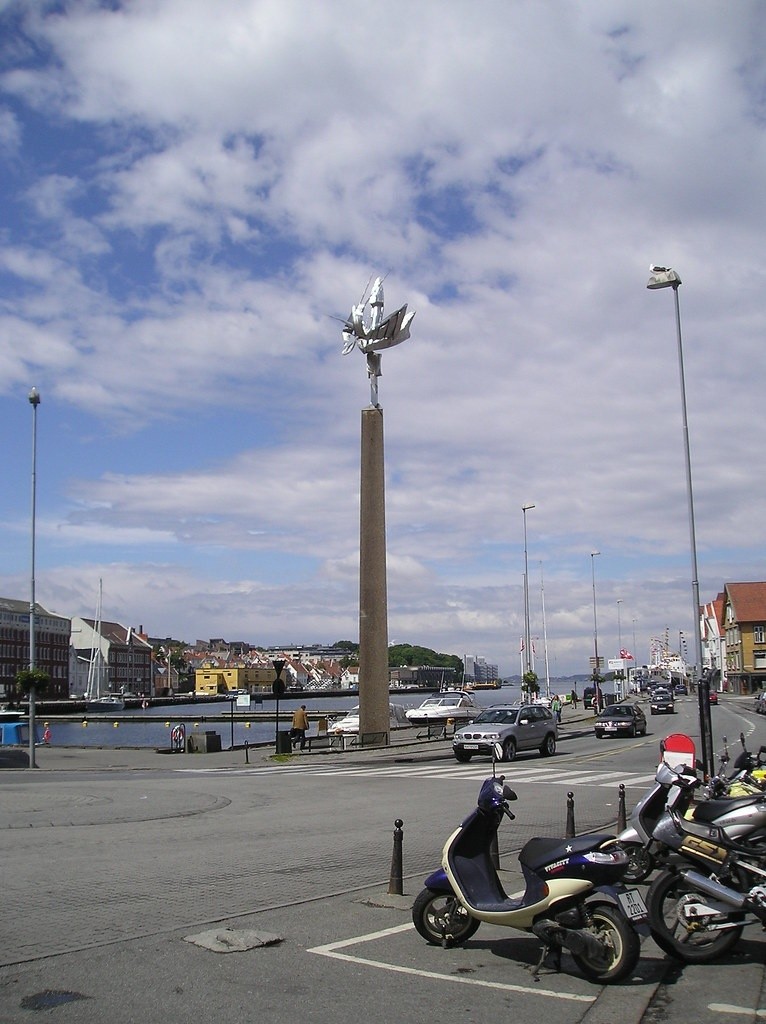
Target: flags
[620,648,633,659]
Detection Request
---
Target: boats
[0,723,41,746]
[650,638,687,677]
[326,701,409,734]
[405,690,486,725]
[514,691,572,708]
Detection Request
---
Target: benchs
[351,731,389,748]
[443,722,469,739]
[301,735,344,754]
[417,726,443,742]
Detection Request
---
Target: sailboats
[85,578,125,711]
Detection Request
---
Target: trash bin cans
[605,693,614,705]
[276,730,291,754]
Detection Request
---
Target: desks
[328,733,359,750]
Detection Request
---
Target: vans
[225,689,248,700]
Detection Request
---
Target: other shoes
[293,741,295,748]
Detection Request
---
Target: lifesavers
[172,726,184,743]
[44,730,52,741]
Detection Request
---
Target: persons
[591,696,598,716]
[571,690,577,709]
[293,705,309,750]
[552,695,563,723]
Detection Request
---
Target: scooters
[409,743,640,984]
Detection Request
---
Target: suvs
[453,703,558,765]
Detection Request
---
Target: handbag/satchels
[290,728,295,738]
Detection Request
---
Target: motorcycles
[615,731,766,964]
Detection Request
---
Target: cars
[584,687,646,739]
[647,680,687,713]
[709,691,718,705]
[754,691,766,716]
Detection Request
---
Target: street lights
[632,619,637,676]
[24,383,41,770]
[521,504,539,702]
[617,599,624,658]
[591,551,602,715]
[647,263,716,778]
